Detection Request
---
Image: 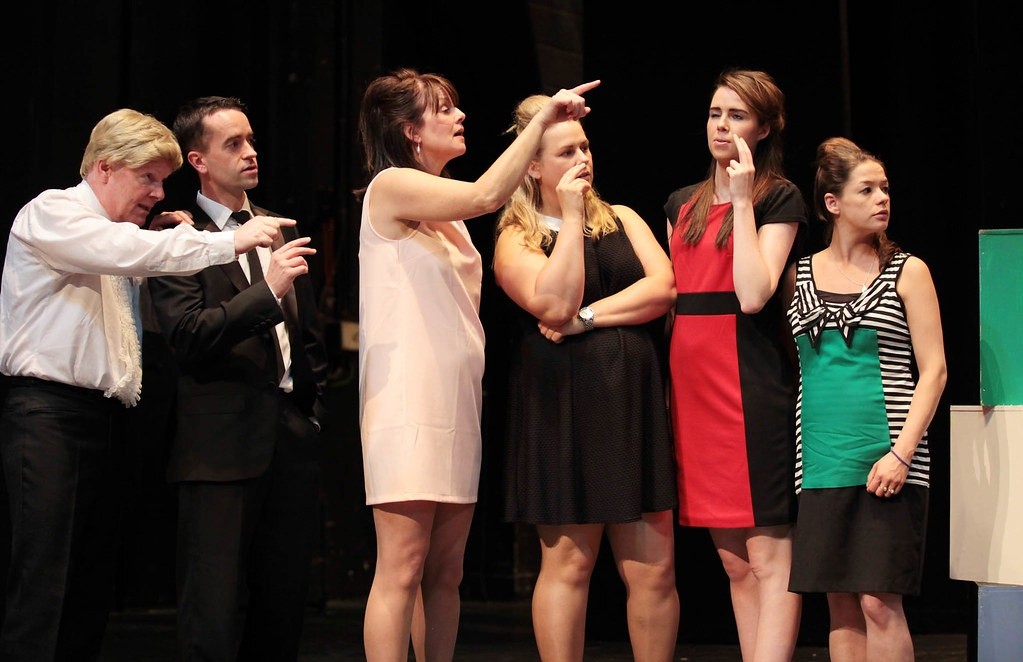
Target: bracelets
[891,447,912,469]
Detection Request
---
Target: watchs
[577,306,595,331]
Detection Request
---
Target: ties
[229,210,285,386]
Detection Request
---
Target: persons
[146,94,317,661]
[664,70,812,662]
[492,93,681,661]
[781,136,950,661]
[357,68,602,662]
[0,108,298,662]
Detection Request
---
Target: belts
[2,372,81,392]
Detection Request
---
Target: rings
[274,217,277,221]
[879,486,894,494]
